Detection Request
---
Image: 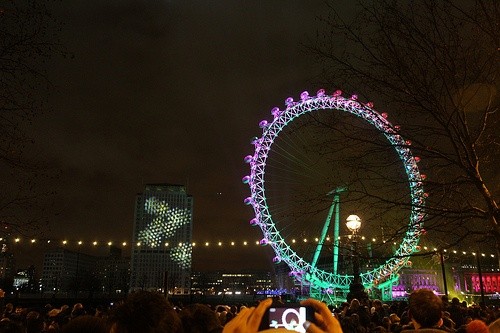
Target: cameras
[257,302,316,332]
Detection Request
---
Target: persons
[0,286,500,333]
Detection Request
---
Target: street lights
[346,212,367,303]
